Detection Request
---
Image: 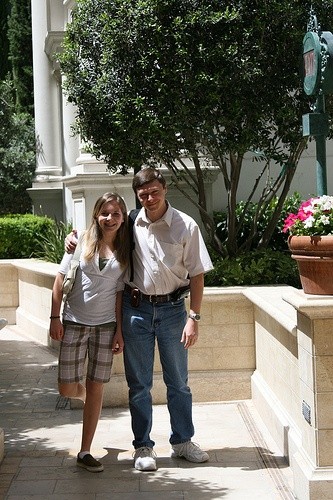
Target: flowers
[282,194,333,235]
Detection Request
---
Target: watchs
[189,313,201,322]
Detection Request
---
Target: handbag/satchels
[62,260,80,294]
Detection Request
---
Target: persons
[49,192,130,472]
[64,169,215,471]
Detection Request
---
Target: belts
[124,283,182,303]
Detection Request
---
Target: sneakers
[132,447,157,470]
[171,441,209,462]
[77,452,104,471]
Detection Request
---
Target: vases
[288,235,333,295]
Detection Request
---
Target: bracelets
[50,316,60,319]
